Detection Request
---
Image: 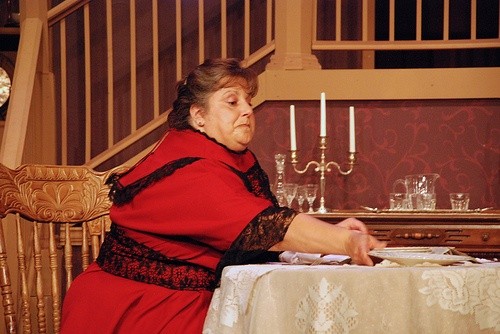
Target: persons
[58,58,387,334]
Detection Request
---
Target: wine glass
[295,185,306,214]
[282,184,297,208]
[304,184,319,214]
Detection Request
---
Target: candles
[349,106,355,152]
[320,92,326,135]
[290,105,296,150]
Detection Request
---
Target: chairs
[0,160,132,334]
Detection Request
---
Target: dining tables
[202,259,500,334]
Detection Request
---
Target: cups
[450,193,470,210]
[409,194,436,210]
[390,193,407,210]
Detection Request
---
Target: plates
[371,250,475,266]
[385,247,456,254]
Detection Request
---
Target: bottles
[272,154,287,208]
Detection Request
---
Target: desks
[304,208,500,265]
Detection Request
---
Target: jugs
[393,174,440,209]
